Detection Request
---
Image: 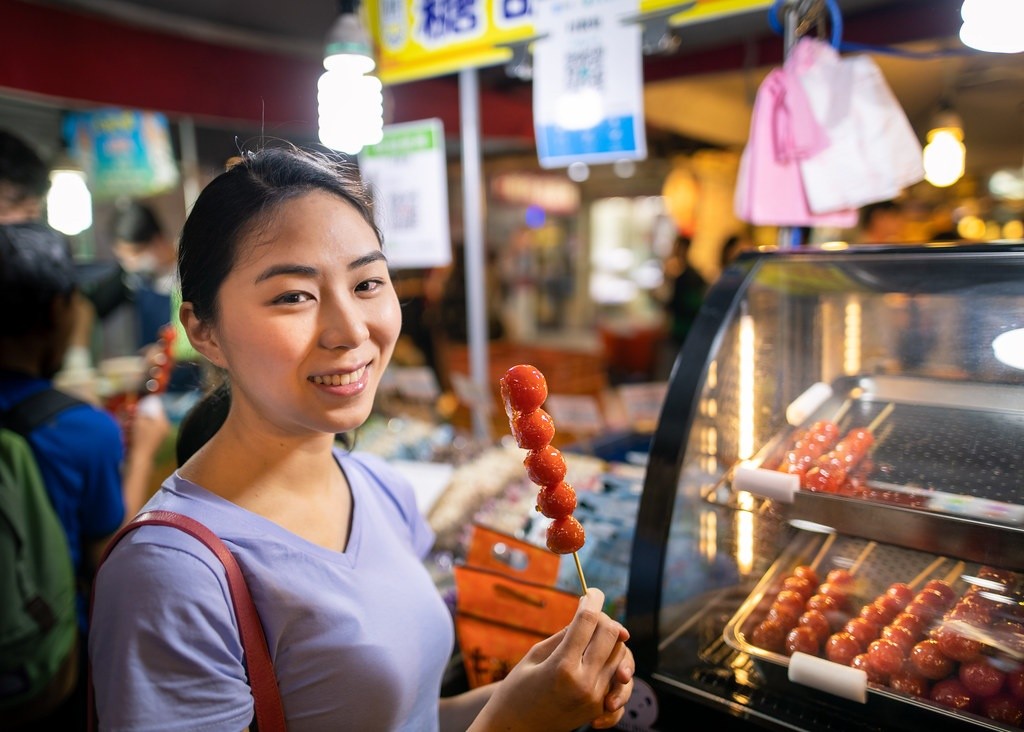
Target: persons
[650,235,708,343]
[0,125,169,732]
[91,148,635,732]
[111,204,202,397]
